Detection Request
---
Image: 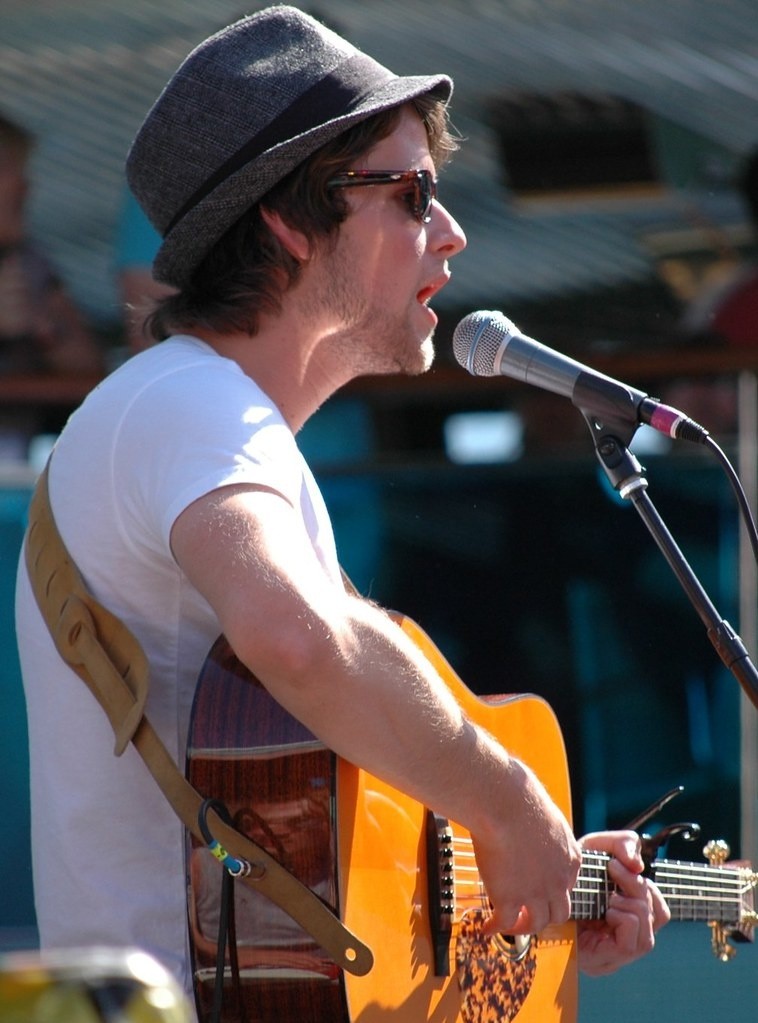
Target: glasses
[326,169,438,224]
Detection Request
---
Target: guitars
[181,603,756,1022]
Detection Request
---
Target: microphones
[453,310,710,445]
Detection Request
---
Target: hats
[125,3,455,291]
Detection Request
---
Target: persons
[12,2,676,1023]
